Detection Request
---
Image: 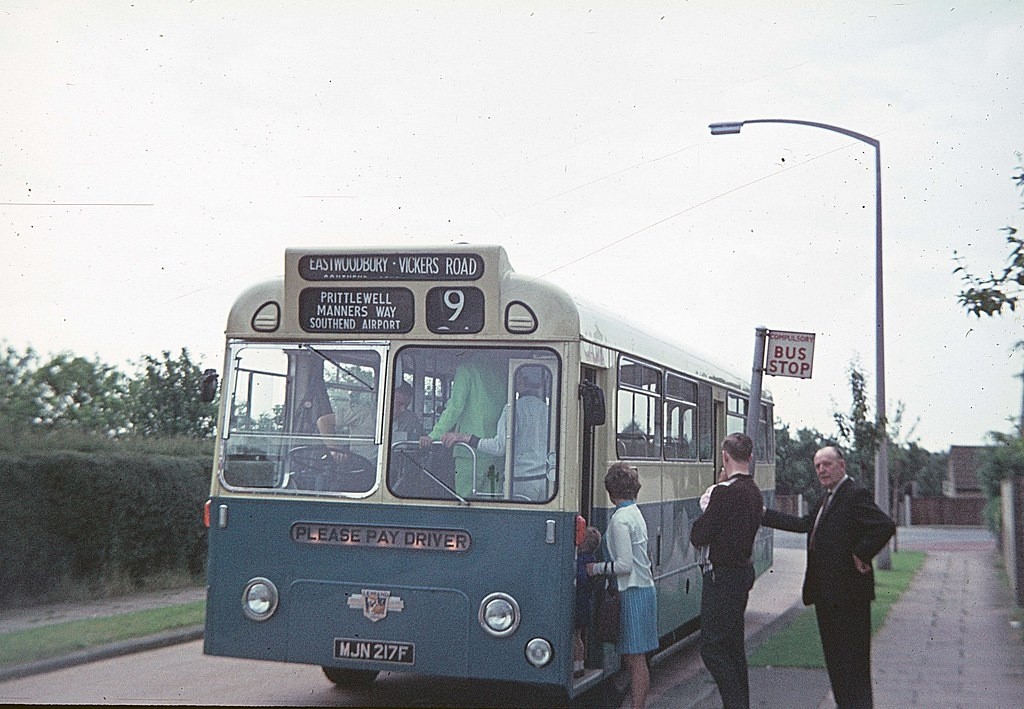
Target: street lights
[709,118,895,571]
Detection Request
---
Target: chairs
[617,433,691,459]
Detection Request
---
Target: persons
[689,432,764,709]
[440,364,550,502]
[761,445,897,709]
[586,462,660,709]
[699,466,731,572]
[571,526,601,679]
[419,349,508,500]
[316,379,428,499]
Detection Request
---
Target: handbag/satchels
[599,562,622,645]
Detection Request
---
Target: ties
[810,493,831,550]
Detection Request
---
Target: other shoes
[573,669,585,678]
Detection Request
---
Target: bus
[202,242,778,709]
